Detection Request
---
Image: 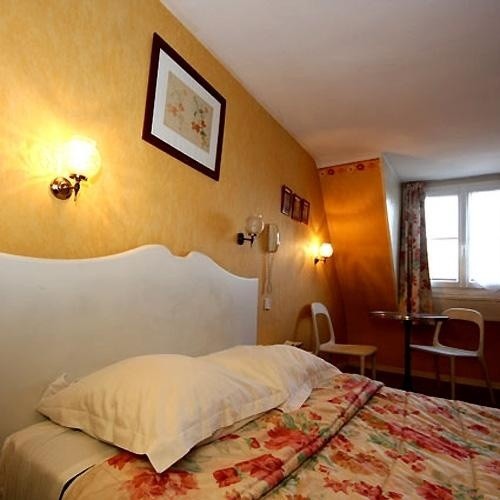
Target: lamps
[49,134,99,200]
[314,243,333,263]
[237,217,264,245]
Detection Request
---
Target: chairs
[310,301,378,381]
[409,307,492,401]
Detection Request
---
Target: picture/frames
[141,32,226,181]
[282,184,310,225]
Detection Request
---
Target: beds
[0,246,500,500]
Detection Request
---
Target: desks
[368,310,448,394]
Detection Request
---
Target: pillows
[196,344,343,411]
[37,354,287,473]
